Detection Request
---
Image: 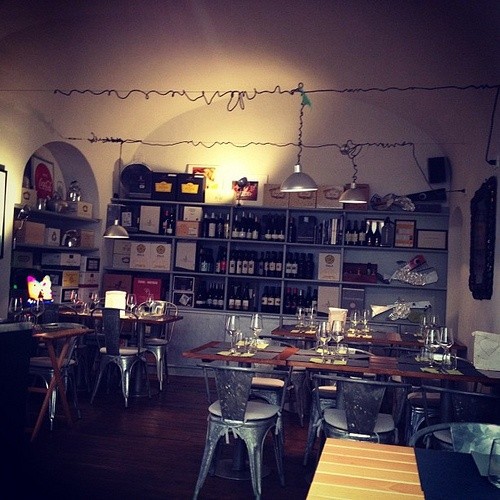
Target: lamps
[101,141,130,238]
[338,157,368,204]
[280,100,320,192]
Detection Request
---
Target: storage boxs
[14,188,101,306]
[101,273,164,306]
[112,238,197,272]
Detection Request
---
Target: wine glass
[70,290,155,318]
[291,305,373,364]
[8,296,45,331]
[416,314,457,371]
[224,313,264,357]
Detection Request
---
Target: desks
[25,328,94,451]
[182,340,300,480]
[57,307,183,398]
[385,330,467,350]
[306,437,424,500]
[272,325,390,430]
[398,357,500,422]
[287,348,400,483]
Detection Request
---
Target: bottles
[161,209,394,317]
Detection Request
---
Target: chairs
[192,325,500,500]
[15,300,180,431]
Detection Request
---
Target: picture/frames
[230,173,268,206]
[188,164,223,194]
[30,154,54,200]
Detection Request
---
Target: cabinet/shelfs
[101,199,449,378]
[11,203,101,315]
